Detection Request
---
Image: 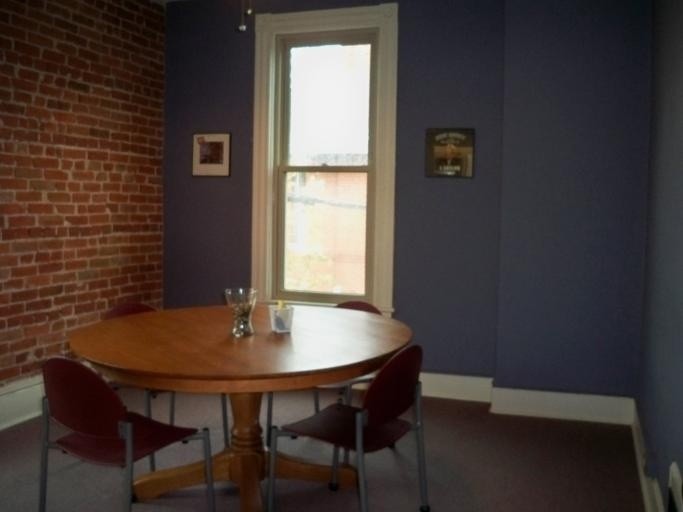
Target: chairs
[312,299,380,415]
[36,356,217,510]
[265,344,430,511]
[98,301,177,429]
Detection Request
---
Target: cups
[269,305,293,333]
[225,288,258,337]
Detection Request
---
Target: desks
[68,302,412,510]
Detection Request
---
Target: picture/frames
[422,126,475,180]
[190,132,230,178]
